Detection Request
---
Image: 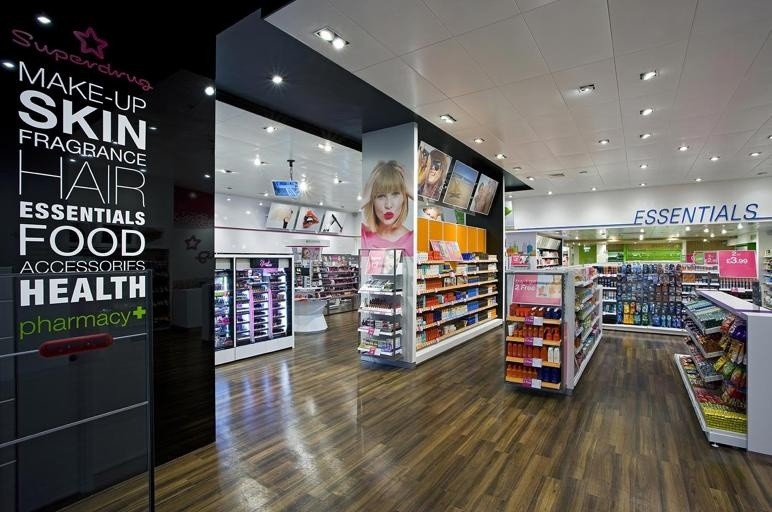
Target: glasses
[432,160,442,171]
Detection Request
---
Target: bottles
[507,304,561,384]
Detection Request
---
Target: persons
[418,149,447,200]
[418,146,430,186]
[423,208,442,220]
[362,161,413,256]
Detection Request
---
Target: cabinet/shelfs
[215,254,294,366]
[673,289,772,458]
[356,248,405,360]
[295,257,358,316]
[417,260,504,364]
[504,269,604,394]
